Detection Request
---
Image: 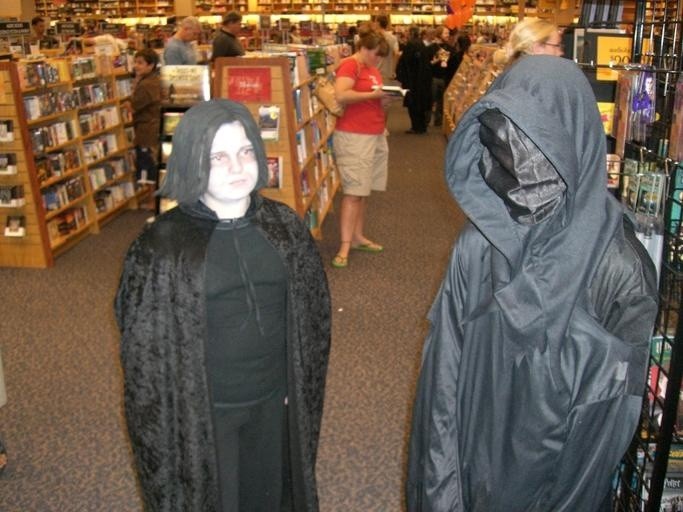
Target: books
[28,51,141,245]
[371,86,409,95]
[246,41,352,230]
[258,105,280,141]
[159,169,167,188]
[160,64,211,104]
[162,112,186,136]
[162,142,173,163]
[159,198,178,215]
[264,157,282,190]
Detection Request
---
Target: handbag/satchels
[312,75,346,118]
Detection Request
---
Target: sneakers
[145,216,156,223]
[405,129,422,134]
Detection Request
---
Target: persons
[331,20,388,266]
[120,48,162,224]
[0,357,8,472]
[493,17,563,63]
[29,16,58,53]
[288,26,303,45]
[395,24,497,135]
[209,13,246,70]
[64,16,98,55]
[164,16,202,66]
[337,17,401,137]
[632,65,654,125]
[114,97,333,512]
[68,18,127,54]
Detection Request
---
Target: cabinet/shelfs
[216,42,344,236]
[0,47,163,272]
[39,2,578,143]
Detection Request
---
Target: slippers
[331,253,349,267]
[351,240,384,253]
